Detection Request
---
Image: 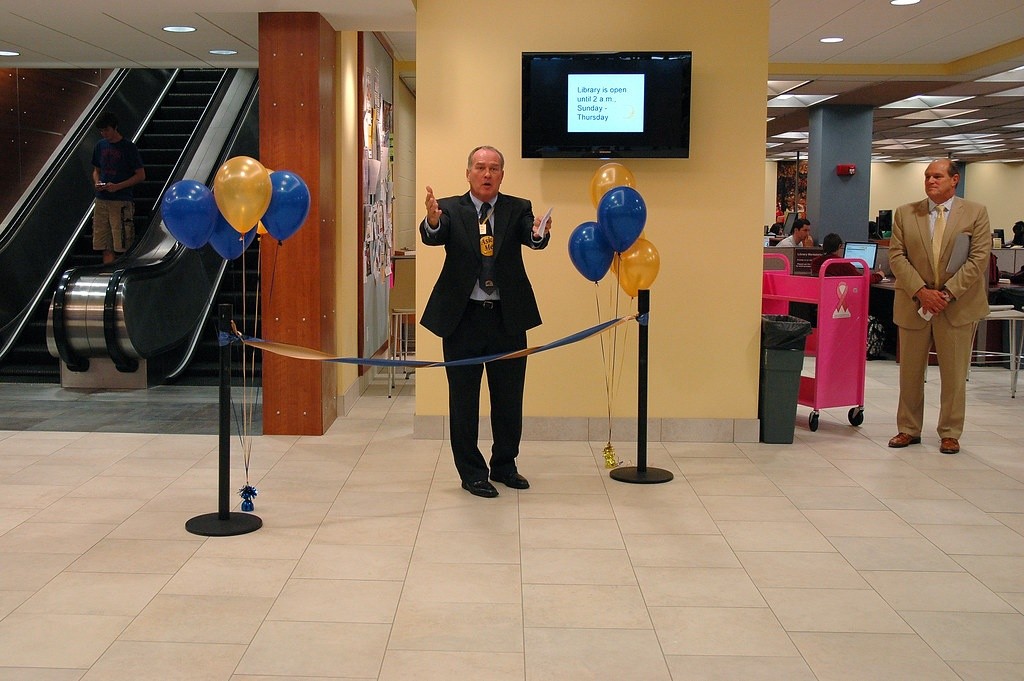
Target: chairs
[924,305,1024,398]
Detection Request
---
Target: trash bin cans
[757,312,811,443]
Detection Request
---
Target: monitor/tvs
[522,51,692,160]
[842,242,878,271]
[994,229,1005,244]
[783,213,799,235]
[763,235,776,247]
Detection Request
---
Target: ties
[932,206,946,289]
[478,202,497,295]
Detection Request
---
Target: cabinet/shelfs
[762,253,870,432]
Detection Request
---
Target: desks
[869,273,896,291]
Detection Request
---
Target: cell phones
[97,183,107,187]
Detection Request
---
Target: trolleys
[762,252,869,431]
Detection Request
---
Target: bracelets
[941,291,951,301]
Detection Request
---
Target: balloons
[566,162,660,299]
[160,155,310,266]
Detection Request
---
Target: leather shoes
[462,479,499,498]
[888,432,921,447]
[939,437,960,452]
[490,470,530,489]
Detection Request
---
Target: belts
[470,299,500,309]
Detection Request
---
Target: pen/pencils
[878,264,881,271]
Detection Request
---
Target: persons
[764,219,814,246]
[419,146,553,498]
[887,157,992,453]
[811,232,885,283]
[92,113,146,264]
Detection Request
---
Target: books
[395,250,416,255]
